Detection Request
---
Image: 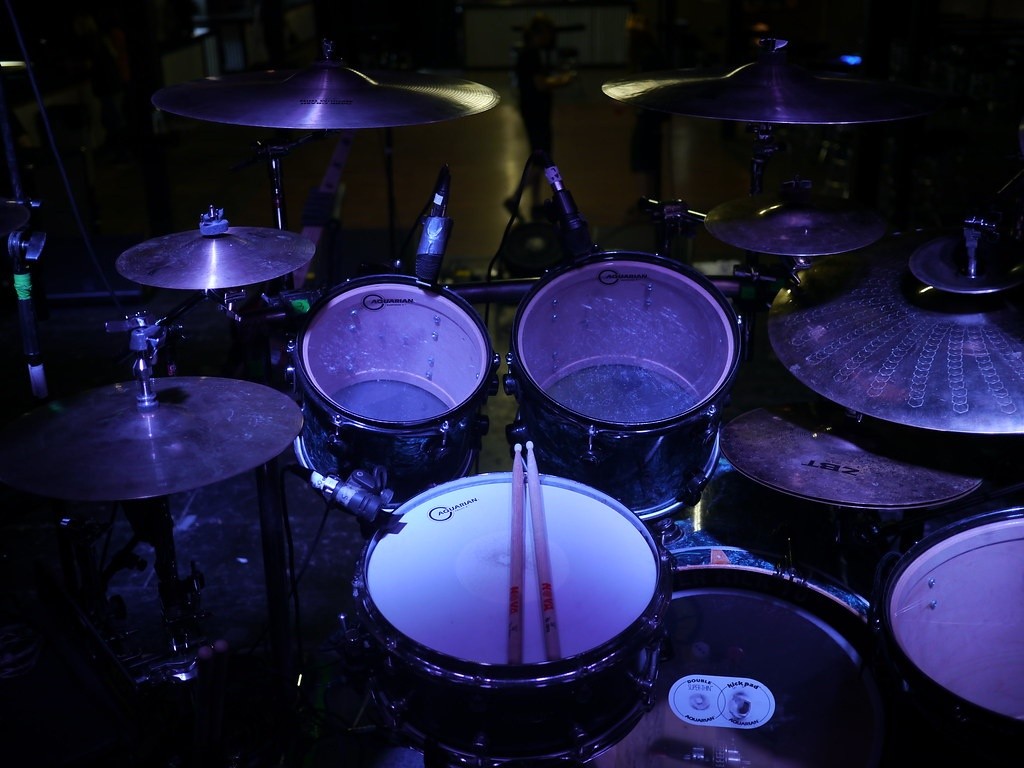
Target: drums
[585,456,924,768]
[356,470,671,768]
[882,506,1024,768]
[292,274,494,509]
[510,250,743,523]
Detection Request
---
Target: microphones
[287,464,384,523]
[539,152,593,253]
[414,169,452,283]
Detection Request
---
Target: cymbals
[767,230,1024,436]
[703,194,889,258]
[600,62,940,124]
[718,400,985,510]
[0,376,307,502]
[150,63,501,130]
[114,226,317,291]
